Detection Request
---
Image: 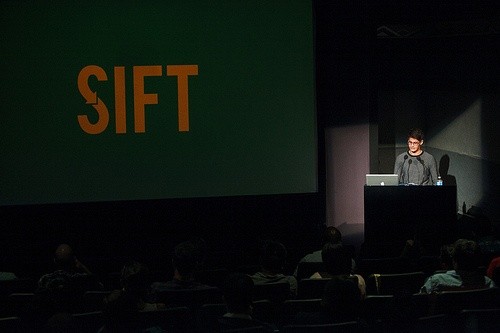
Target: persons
[394,129,438,186]
[0,226,500,333]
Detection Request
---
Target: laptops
[366,174,398,186]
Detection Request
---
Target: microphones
[399,155,408,184]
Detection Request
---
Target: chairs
[0,245,500,333]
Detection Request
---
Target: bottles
[436,176,443,186]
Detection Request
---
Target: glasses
[408,142,419,144]
